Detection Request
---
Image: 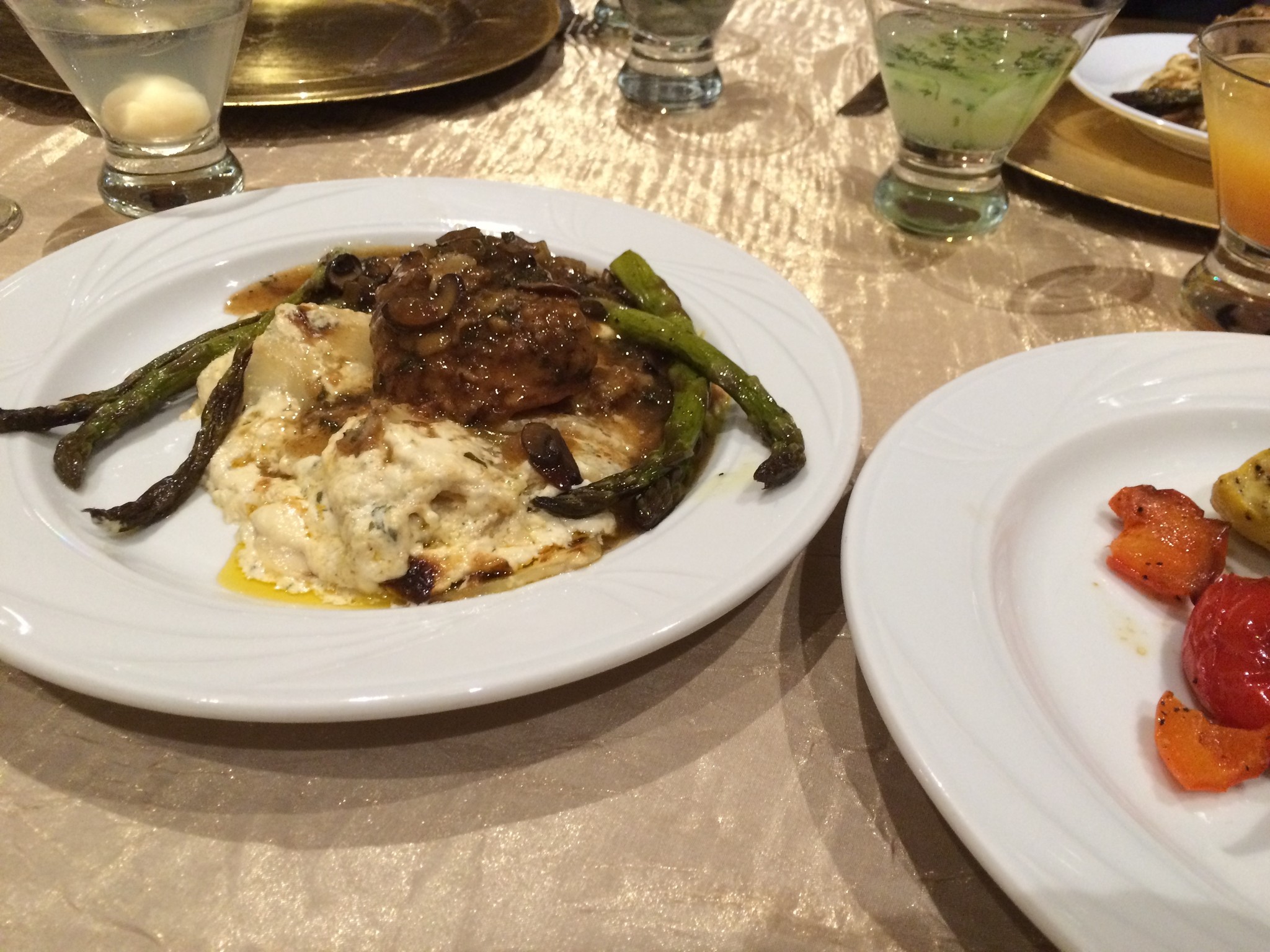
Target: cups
[614,1,738,112]
[13,0,254,219]
[1178,19,1270,334]
[864,1,1128,234]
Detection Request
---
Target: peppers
[1154,691,1270,791]
[1107,485,1229,596]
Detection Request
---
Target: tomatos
[1181,574,1270,731]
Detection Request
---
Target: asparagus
[0,247,347,531]
[530,250,806,525]
[1111,87,1203,115]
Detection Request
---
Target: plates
[0,0,566,107]
[838,330,1269,952]
[0,174,863,726]
[1001,20,1218,230]
[1069,33,1210,160]
